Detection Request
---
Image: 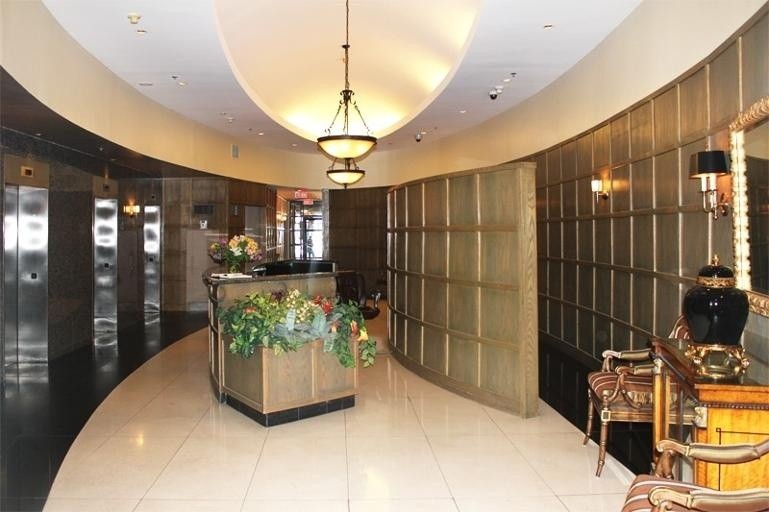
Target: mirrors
[728,94,769,320]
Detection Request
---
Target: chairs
[336,270,380,319]
[621,438,769,512]
[583,286,699,476]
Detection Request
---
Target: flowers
[222,286,378,368]
[208,238,229,265]
[225,234,262,272]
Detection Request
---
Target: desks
[649,333,769,490]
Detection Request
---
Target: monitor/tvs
[271,264,289,272]
[291,261,333,274]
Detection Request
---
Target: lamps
[123,205,140,218]
[316,0,377,192]
[687,150,730,219]
[591,179,608,202]
